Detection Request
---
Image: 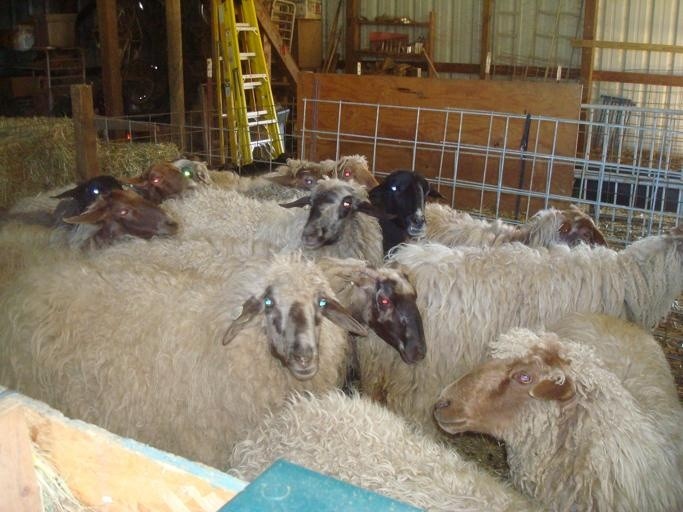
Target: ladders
[214,0,286,176]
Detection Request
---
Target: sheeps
[0,153,683,507]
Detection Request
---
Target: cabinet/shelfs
[291,17,322,68]
[351,10,438,79]
[8,46,87,118]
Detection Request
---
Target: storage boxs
[294,1,324,19]
[32,12,78,47]
